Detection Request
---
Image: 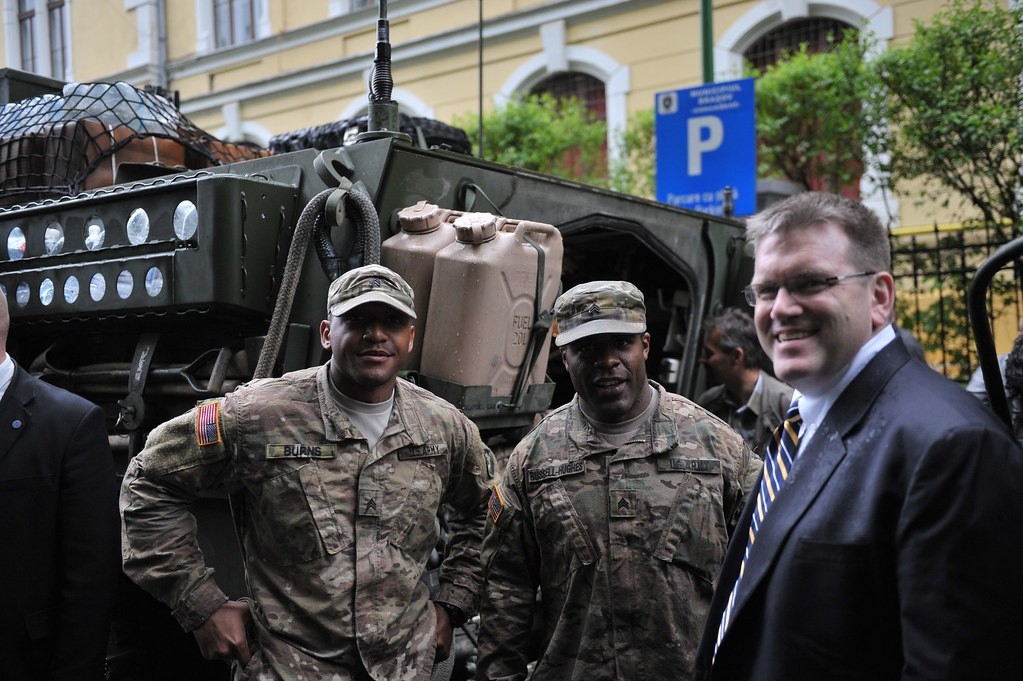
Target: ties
[709,400,804,665]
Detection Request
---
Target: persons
[0,285,122,681]
[118,264,500,681]
[696,309,794,460]
[692,192,1023,681]
[477,281,763,681]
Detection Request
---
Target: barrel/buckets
[418,212,565,398]
[380,201,546,372]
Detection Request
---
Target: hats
[325,262,418,320]
[554,281,647,346]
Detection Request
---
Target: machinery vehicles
[1,1,774,484]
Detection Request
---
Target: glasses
[739,271,876,307]
